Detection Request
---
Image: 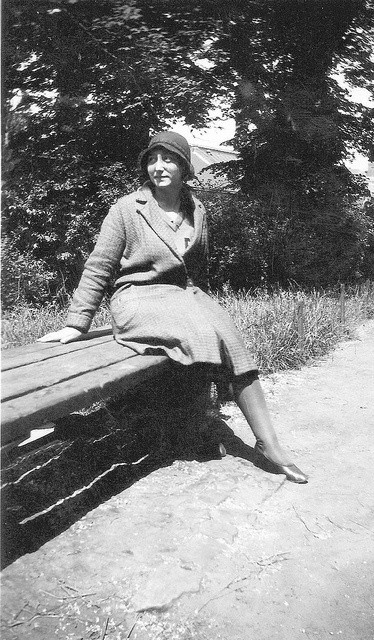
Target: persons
[37,131,309,484]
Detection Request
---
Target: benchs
[1,325,174,446]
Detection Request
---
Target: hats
[137,130,194,184]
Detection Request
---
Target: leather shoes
[254,441,309,484]
